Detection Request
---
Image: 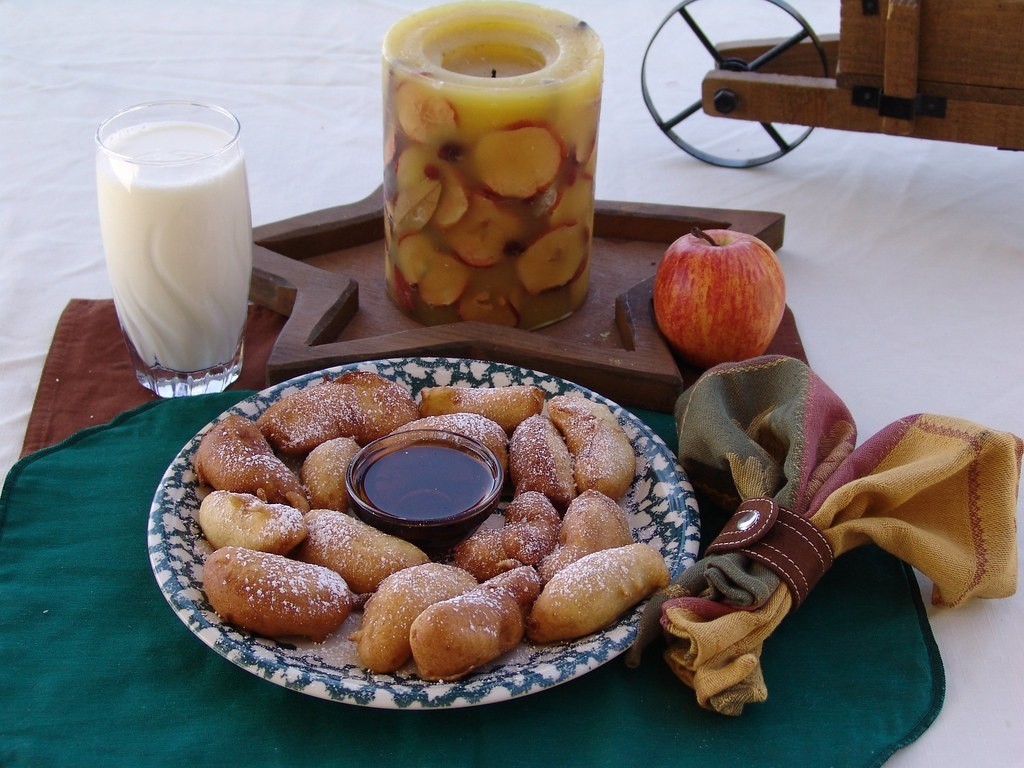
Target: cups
[94,100,251,399]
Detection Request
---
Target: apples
[654,226,786,365]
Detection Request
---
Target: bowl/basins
[345,428,504,560]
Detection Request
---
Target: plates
[147,357,700,711]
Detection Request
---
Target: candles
[383,0,604,330]
